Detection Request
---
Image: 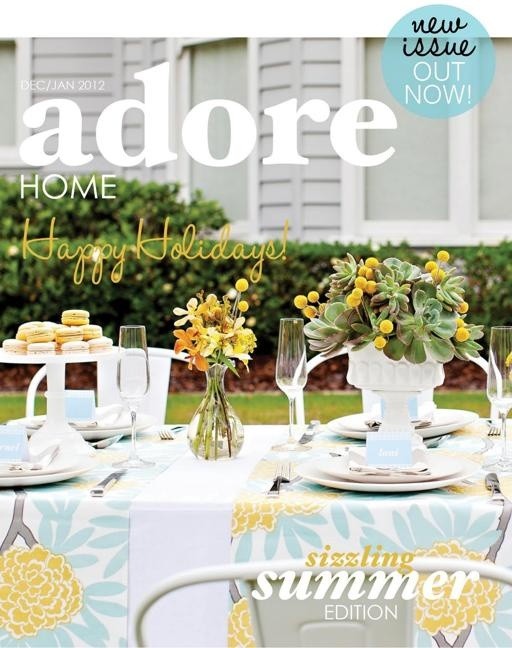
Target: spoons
[91,434,123,450]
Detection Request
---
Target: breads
[3,310,112,357]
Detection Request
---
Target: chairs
[133,558,512,647]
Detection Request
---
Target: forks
[484,419,501,438]
[155,425,185,442]
[268,462,292,495]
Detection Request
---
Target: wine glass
[113,326,155,471]
[486,326,512,474]
[273,319,313,480]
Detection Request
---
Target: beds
[0,419,512,648]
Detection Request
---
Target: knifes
[90,468,129,499]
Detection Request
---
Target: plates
[1,463,92,488]
[4,413,150,442]
[298,407,480,493]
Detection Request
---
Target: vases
[186,362,243,461]
[346,344,446,425]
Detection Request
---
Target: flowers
[169,278,253,376]
[294,251,484,367]
